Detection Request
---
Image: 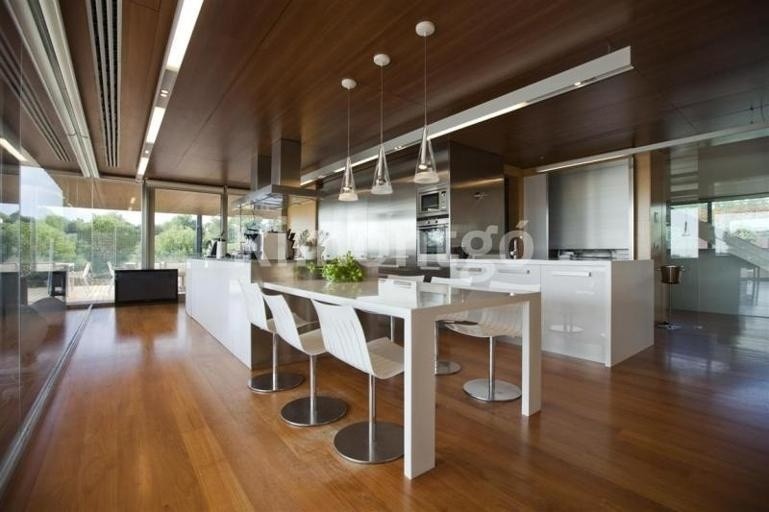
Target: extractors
[228,138,325,214]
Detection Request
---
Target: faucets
[510,239,519,259]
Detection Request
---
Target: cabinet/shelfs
[448,259,493,289]
[540,257,612,368]
[493,260,540,294]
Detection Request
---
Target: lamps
[333,74,361,204]
[409,20,443,186]
[362,51,399,197]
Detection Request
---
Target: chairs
[381,272,426,344]
[260,292,352,430]
[423,276,474,379]
[232,277,321,400]
[56,259,187,297]
[307,295,406,467]
[50,271,67,299]
[443,278,538,405]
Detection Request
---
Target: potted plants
[294,227,330,260]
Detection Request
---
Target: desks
[112,269,179,306]
[255,276,546,482]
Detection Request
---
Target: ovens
[416,183,450,269]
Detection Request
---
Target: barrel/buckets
[655,265,687,286]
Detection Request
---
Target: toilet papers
[216,240,226,259]
